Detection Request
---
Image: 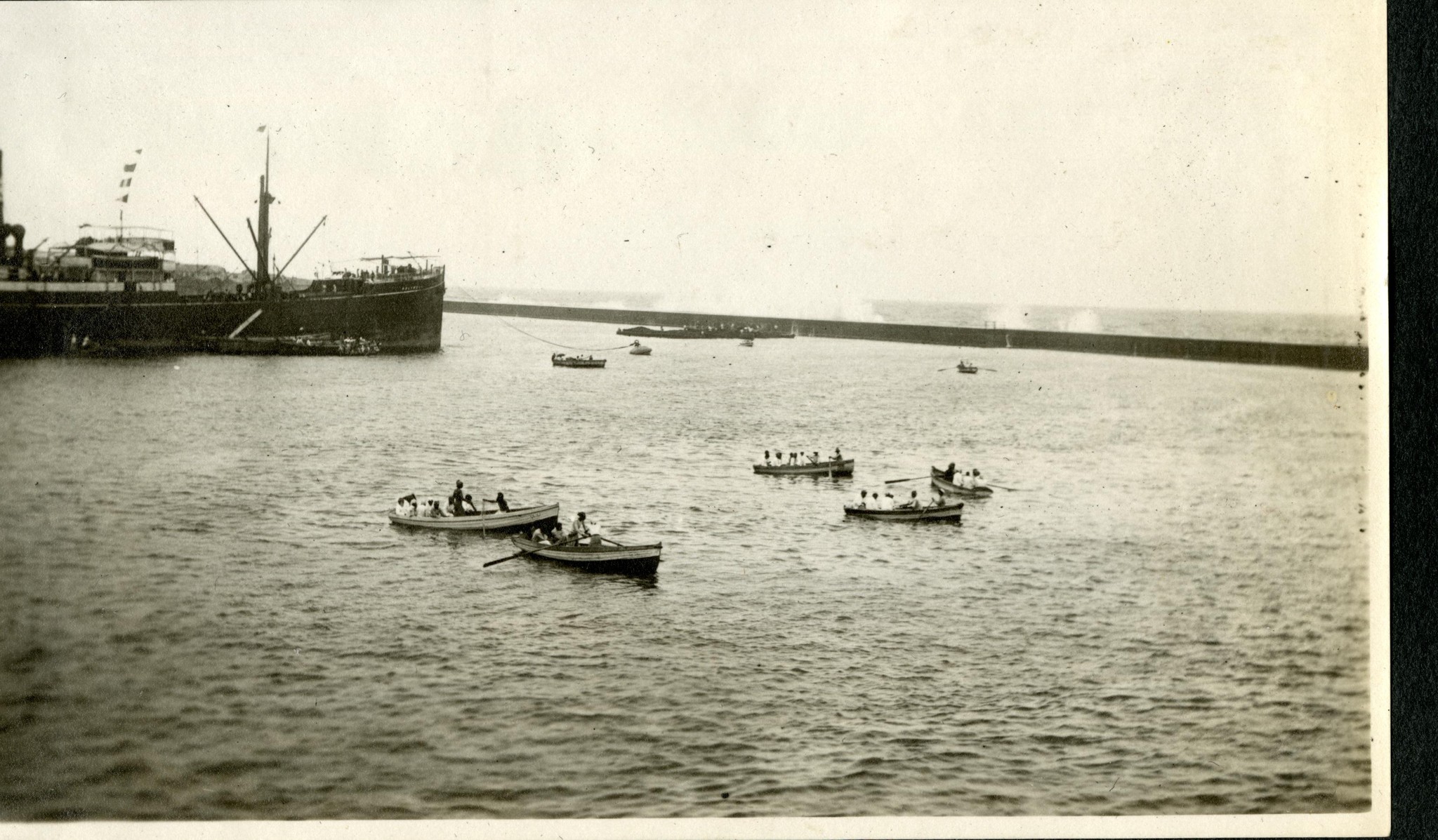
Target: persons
[530,512,591,547]
[395,480,509,518]
[762,448,843,467]
[854,463,986,510]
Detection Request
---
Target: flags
[119,162,136,187]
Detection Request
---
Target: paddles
[601,537,664,563]
[482,500,488,539]
[885,475,947,484]
[829,457,834,480]
[986,483,1018,492]
[482,535,598,568]
[937,366,959,371]
[976,368,997,372]
[806,455,818,467]
[913,498,935,526]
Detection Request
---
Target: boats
[0,121,446,356]
[931,466,993,497]
[751,459,854,477]
[740,342,753,347]
[958,367,978,373]
[388,502,561,534]
[552,353,607,369]
[844,502,964,524]
[511,532,663,574]
